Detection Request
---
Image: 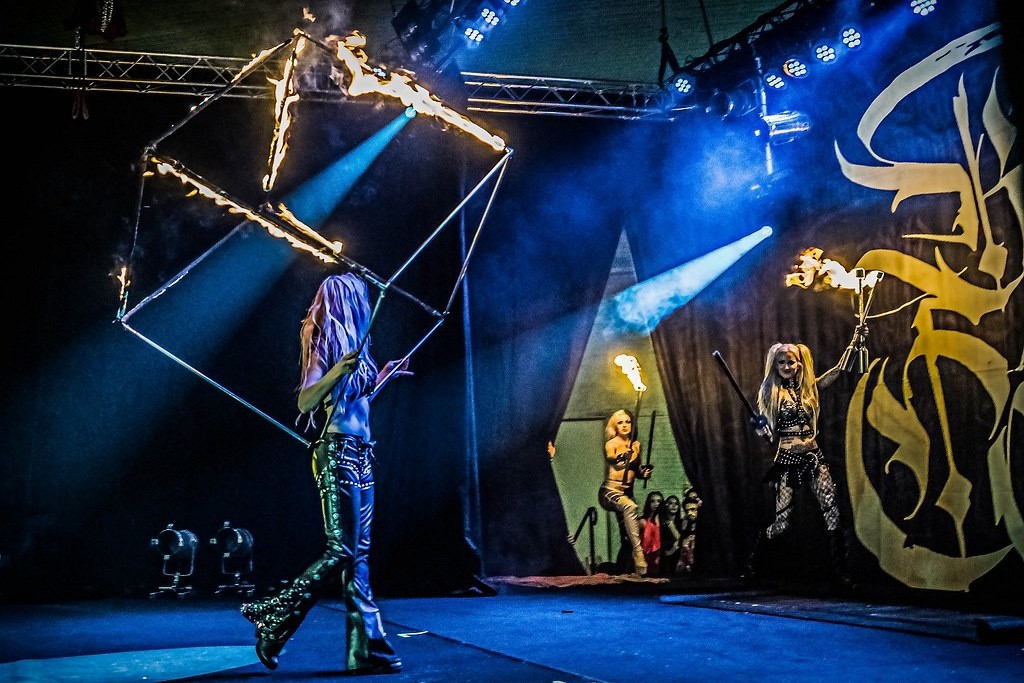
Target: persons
[637,490,664,577]
[658,495,681,578]
[679,497,697,576]
[240,271,404,677]
[598,409,648,576]
[685,488,702,506]
[753,343,858,603]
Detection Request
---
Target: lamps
[810,31,842,67]
[672,69,708,97]
[706,86,758,125]
[839,18,866,52]
[392,1,443,65]
[210,520,259,595]
[784,48,813,82]
[754,112,816,147]
[763,55,791,93]
[143,524,203,599]
[456,1,528,48]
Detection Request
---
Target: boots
[240,575,315,667]
[348,610,405,673]
[741,529,768,577]
[823,529,858,593]
[624,510,649,575]
